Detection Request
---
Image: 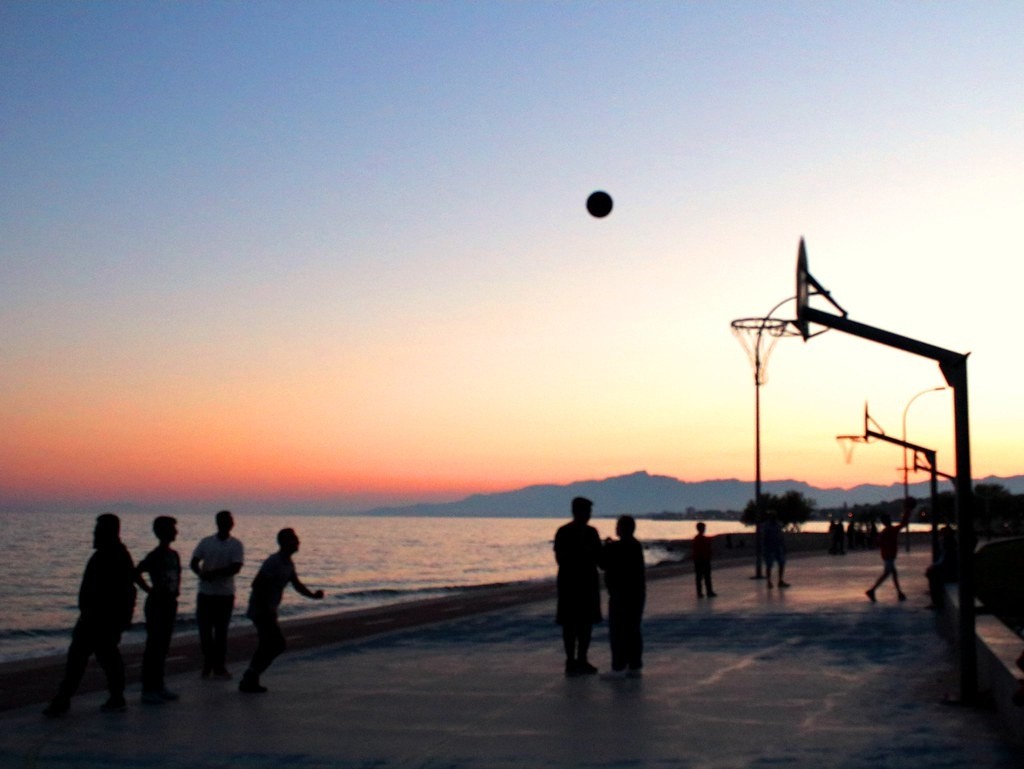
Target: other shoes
[866,590,876,602]
[778,582,789,587]
[239,681,267,693]
[898,594,905,600]
[599,670,626,681]
[213,667,233,679]
[707,593,716,598]
[566,659,597,674]
[626,670,642,678]
[202,661,214,680]
[698,594,703,598]
[42,699,70,718]
[143,687,178,703]
[101,696,128,713]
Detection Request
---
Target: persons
[866,505,912,602]
[690,523,716,598]
[43,513,136,718]
[554,497,613,679]
[135,516,181,705]
[190,511,243,680]
[599,516,647,678]
[829,519,878,554]
[762,510,790,588]
[924,528,959,610]
[240,528,323,693]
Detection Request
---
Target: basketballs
[586,191,613,217]
[903,496,918,511]
